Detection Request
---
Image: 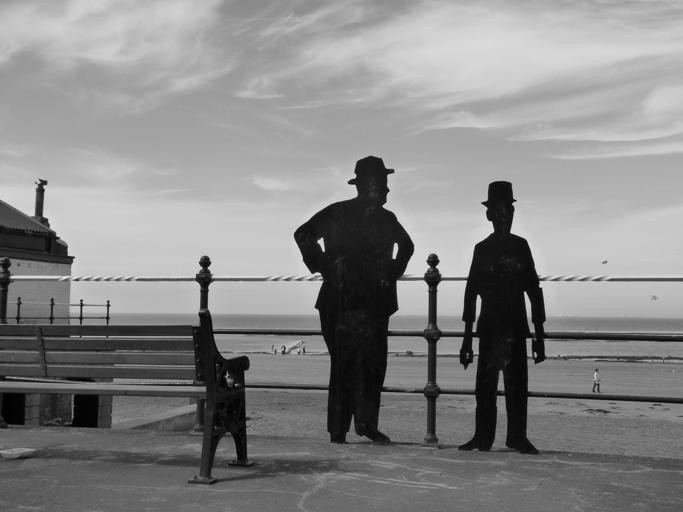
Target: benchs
[1,309,255,484]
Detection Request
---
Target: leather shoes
[458,435,539,454]
[330,424,390,443]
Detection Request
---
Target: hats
[348,156,394,185]
[480,181,517,208]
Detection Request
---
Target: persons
[459,205,546,454]
[271,344,286,356]
[297,345,306,354]
[294,174,414,444]
[592,368,601,393]
